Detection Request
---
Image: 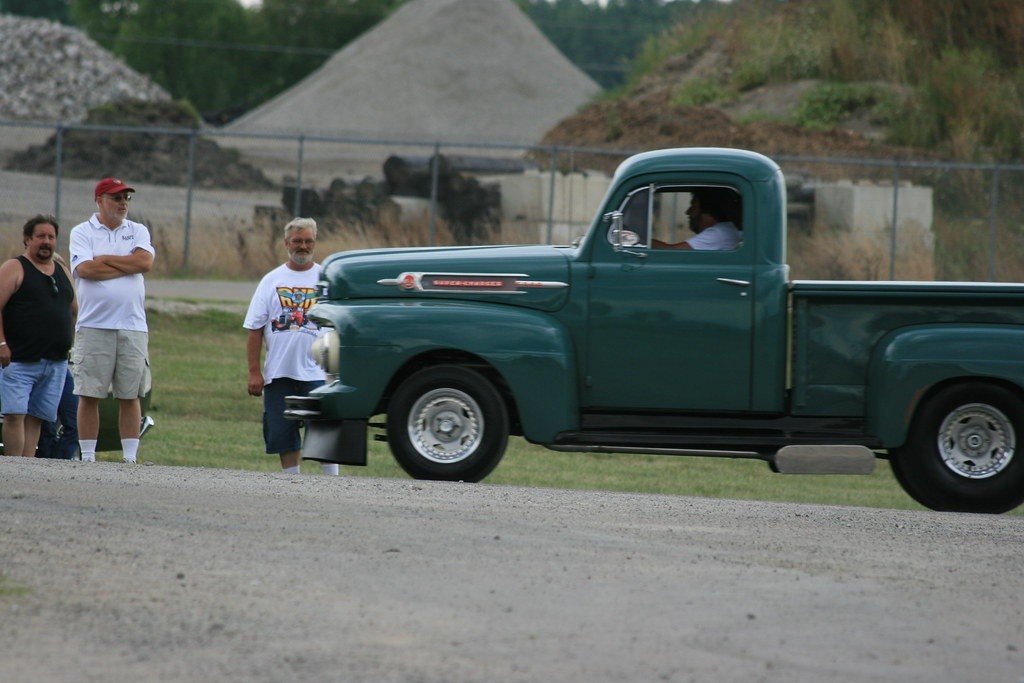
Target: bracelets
[0,342,7,347]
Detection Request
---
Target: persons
[0,214,82,459]
[242,218,339,478]
[69,177,156,465]
[623,186,744,254]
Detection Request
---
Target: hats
[95,178,135,202]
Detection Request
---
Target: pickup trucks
[282,148,1024,516]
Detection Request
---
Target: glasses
[102,194,131,202]
[49,275,58,294]
